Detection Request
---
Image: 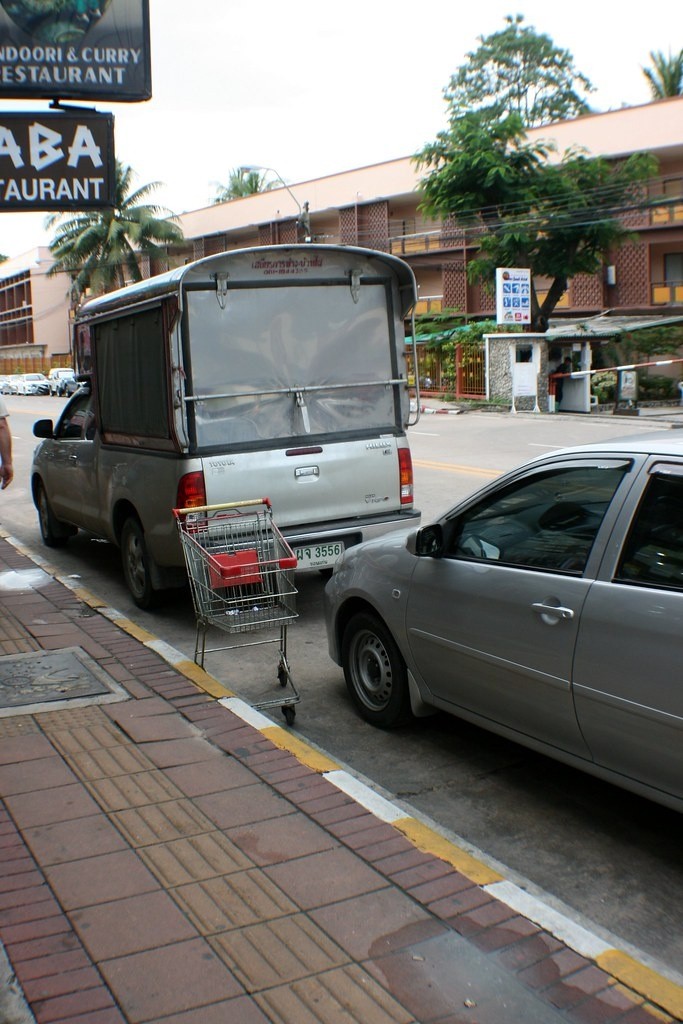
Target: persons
[0,395,14,490]
[555,356,572,402]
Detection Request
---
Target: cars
[0,368,86,398]
[325,427,682,820]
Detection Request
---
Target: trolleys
[171,497,302,728]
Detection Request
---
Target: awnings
[546,315,683,341]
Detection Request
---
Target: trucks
[28,243,421,613]
